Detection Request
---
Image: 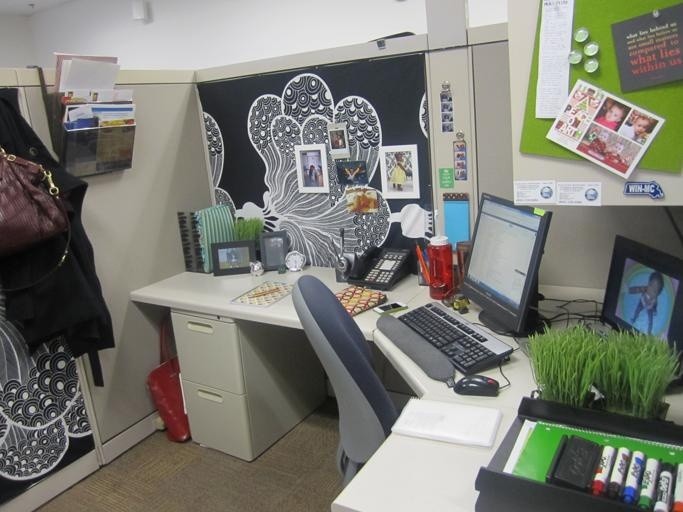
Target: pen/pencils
[414,239,430,285]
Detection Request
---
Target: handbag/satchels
[0,147,75,294]
[148,311,191,444]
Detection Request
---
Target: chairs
[290,274,398,485]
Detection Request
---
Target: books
[500,418,682,491]
[228,281,291,308]
[331,286,386,315]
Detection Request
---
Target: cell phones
[373,301,408,314]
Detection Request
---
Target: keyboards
[398,301,514,377]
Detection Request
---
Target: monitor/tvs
[452,192,553,336]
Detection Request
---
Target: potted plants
[232,216,264,260]
[526,319,606,409]
[598,329,681,424]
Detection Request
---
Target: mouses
[453,374,500,397]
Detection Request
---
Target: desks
[128,264,683,512]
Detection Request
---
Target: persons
[392,153,406,191]
[342,164,362,183]
[574,90,596,119]
[309,166,319,182]
[598,105,624,130]
[626,116,650,139]
[316,167,323,186]
[628,273,665,334]
[337,134,344,148]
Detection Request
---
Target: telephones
[347,246,413,290]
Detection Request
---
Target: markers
[653,462,675,512]
[639,458,661,510]
[592,446,617,497]
[673,463,683,512]
[623,450,646,504]
[609,446,632,498]
